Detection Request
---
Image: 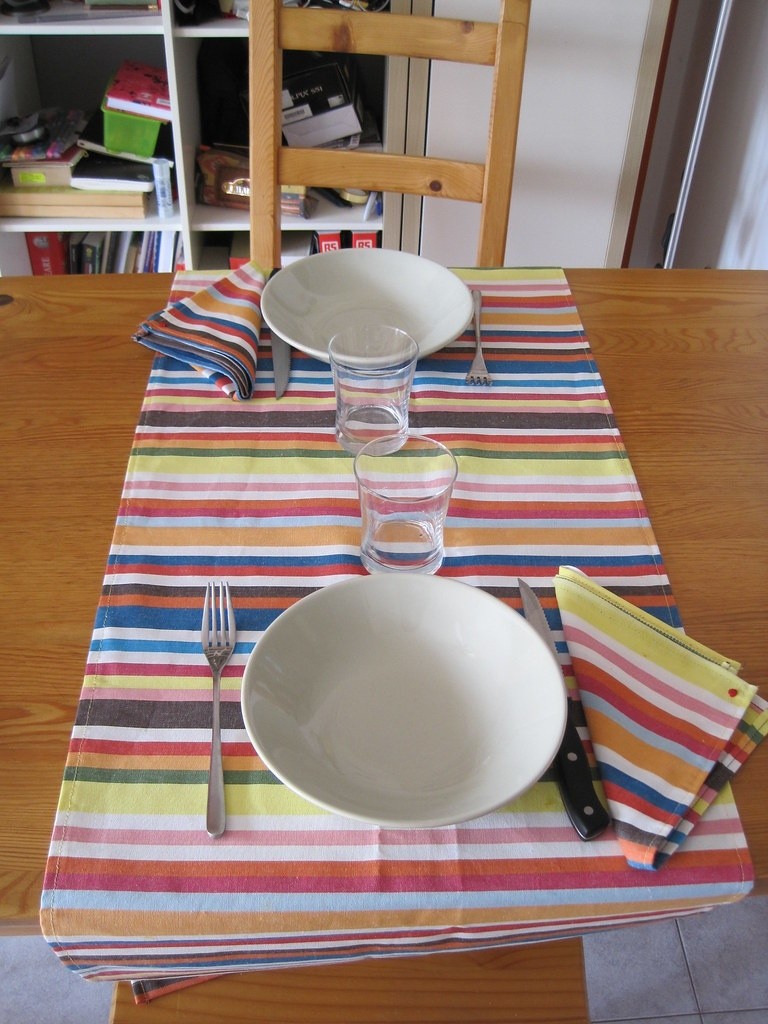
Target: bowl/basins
[238,571,569,829]
[259,247,475,371]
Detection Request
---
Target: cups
[353,434,458,575]
[326,323,422,455]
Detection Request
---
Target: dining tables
[0,269,768,937]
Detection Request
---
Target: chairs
[250,0,532,270]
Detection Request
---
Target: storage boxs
[101,65,167,156]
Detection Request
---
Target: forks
[466,289,494,388]
[201,581,236,839]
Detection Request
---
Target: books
[0,60,173,218]
[26,231,185,276]
[198,230,381,270]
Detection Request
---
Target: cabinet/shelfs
[0,0,403,277]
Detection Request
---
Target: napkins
[552,563,768,870]
[132,257,265,401]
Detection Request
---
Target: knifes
[517,577,611,842]
[267,267,292,401]
